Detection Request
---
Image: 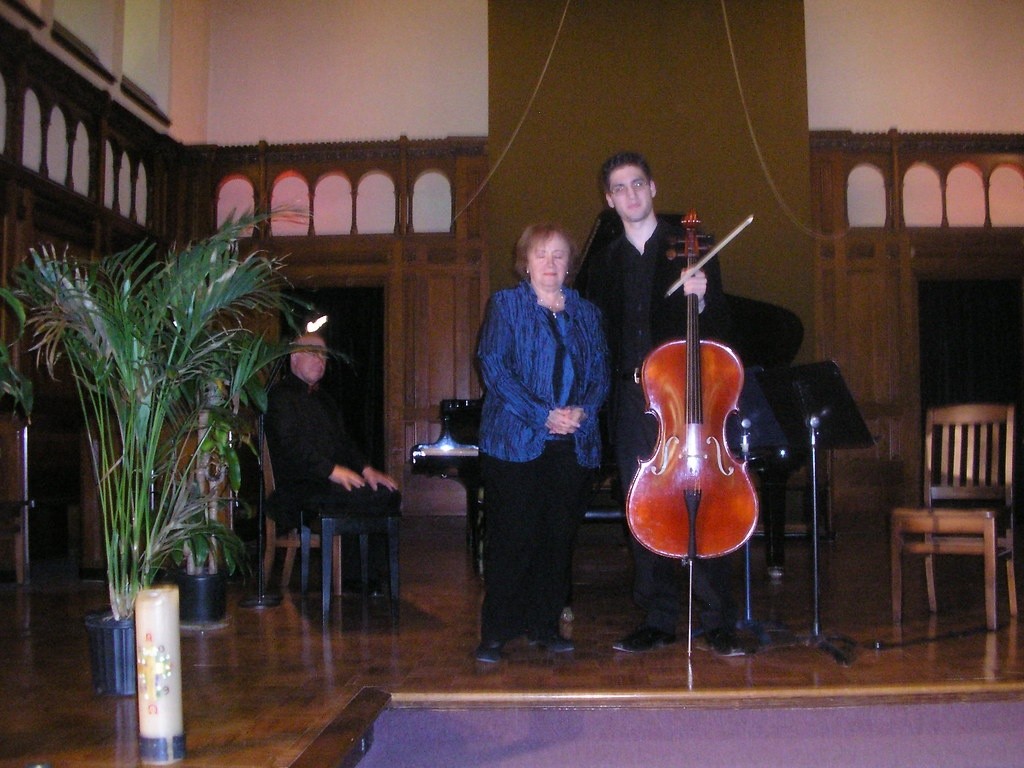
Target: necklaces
[538,289,564,316]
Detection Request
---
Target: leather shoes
[611,623,676,651]
[704,625,746,657]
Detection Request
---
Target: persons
[265,333,400,610]
[583,152,752,657]
[475,223,608,663]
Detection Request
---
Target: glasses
[609,180,650,196]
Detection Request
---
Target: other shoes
[475,635,504,662]
[528,631,575,652]
[331,575,384,597]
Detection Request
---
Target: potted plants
[0,203,355,699]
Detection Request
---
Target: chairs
[253,417,342,600]
[888,402,1017,633]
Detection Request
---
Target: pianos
[406,209,807,601]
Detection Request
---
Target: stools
[300,487,403,616]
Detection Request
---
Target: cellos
[619,209,761,661]
